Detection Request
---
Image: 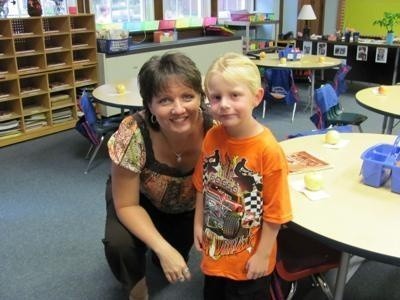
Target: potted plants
[371,11,400,44]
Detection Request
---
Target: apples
[115,84,125,92]
[280,57,287,64]
[304,172,324,190]
[260,52,265,58]
[378,86,386,94]
[326,130,340,144]
[319,57,324,62]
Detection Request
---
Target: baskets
[96,36,133,55]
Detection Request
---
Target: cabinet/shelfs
[0,13,98,149]
[225,19,280,58]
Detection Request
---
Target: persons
[102,49,223,300]
[191,51,293,300]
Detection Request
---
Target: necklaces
[163,132,192,163]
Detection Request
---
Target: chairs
[246,51,399,300]
[81,87,127,175]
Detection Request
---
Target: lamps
[296,5,316,37]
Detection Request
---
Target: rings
[177,276,183,281]
[183,267,189,274]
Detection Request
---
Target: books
[96,28,129,51]
[0,28,96,138]
[286,150,332,174]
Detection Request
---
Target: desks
[92,77,210,117]
[292,37,400,86]
